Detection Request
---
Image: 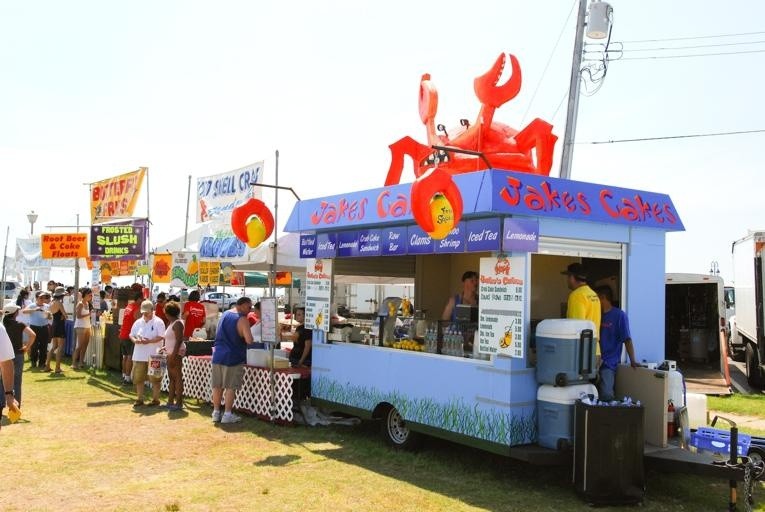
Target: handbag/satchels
[147,349,166,378]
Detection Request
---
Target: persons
[280,307,312,400]
[0,280,185,422]
[561,262,603,371]
[250,301,263,344]
[592,285,648,400]
[441,271,479,321]
[182,291,206,338]
[210,297,253,423]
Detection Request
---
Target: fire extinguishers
[668,399,675,437]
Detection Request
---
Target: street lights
[26,209,39,234]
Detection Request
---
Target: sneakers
[212,411,241,424]
[133,399,183,410]
[123,379,131,385]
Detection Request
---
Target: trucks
[727,230,765,390]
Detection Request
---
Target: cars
[199,292,238,312]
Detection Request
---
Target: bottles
[444,331,465,357]
[425,329,439,354]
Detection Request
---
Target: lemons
[388,302,395,317]
[401,299,410,317]
[393,338,423,351]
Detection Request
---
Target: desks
[102,323,122,371]
[144,356,311,428]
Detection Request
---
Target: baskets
[690,427,751,456]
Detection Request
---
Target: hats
[52,286,69,297]
[3,303,21,315]
[462,271,475,281]
[140,301,154,313]
[561,263,587,281]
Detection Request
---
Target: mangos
[428,193,453,240]
[246,217,264,247]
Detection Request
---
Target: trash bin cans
[575,397,647,508]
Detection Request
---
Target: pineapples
[187,254,198,275]
